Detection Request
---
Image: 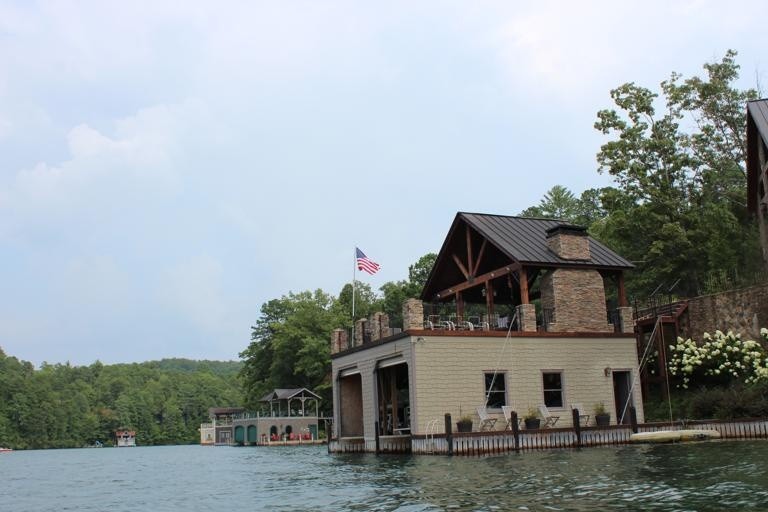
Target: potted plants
[523,405,543,430]
[455,413,474,432]
[591,400,611,426]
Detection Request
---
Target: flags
[356,247,382,275]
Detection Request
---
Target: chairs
[571,403,591,427]
[536,404,560,428]
[423,314,490,332]
[393,428,411,435]
[501,406,522,431]
[475,406,498,432]
